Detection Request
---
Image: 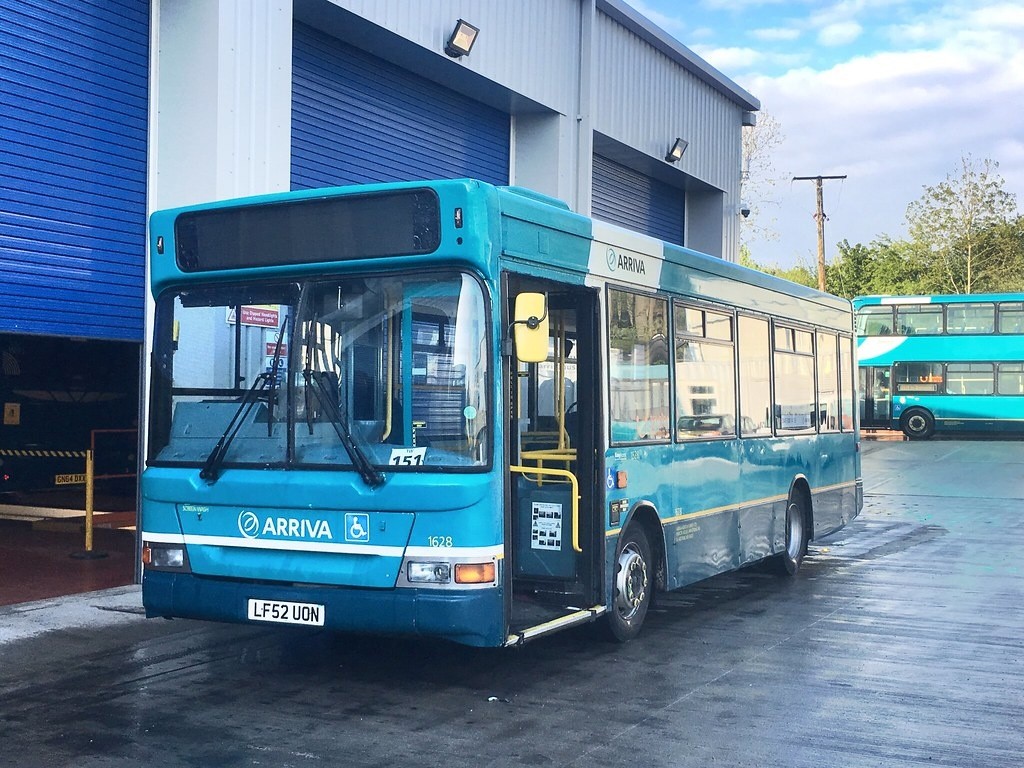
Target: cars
[0,427,100,505]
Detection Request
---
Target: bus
[842,291,1024,440]
[131,178,862,651]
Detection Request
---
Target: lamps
[444,18,480,59]
[665,138,689,162]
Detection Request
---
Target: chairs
[875,373,1024,395]
[876,318,1024,335]
[284,376,850,481]
